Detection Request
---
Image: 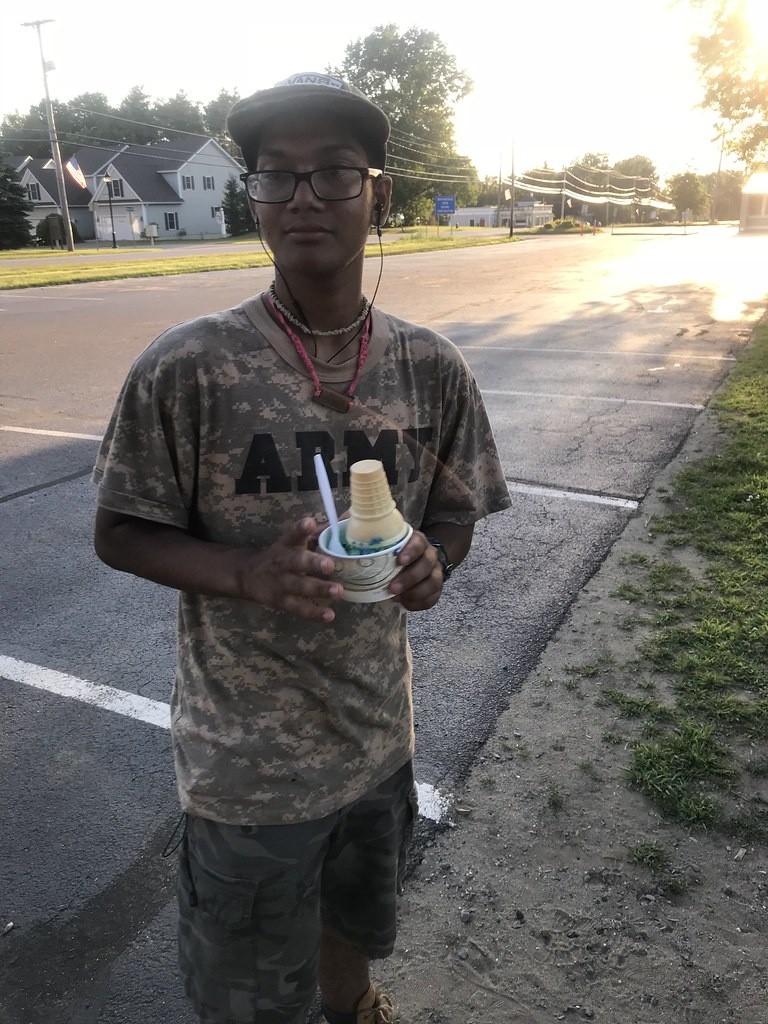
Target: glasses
[239,165,384,205]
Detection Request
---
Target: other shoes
[323,982,393,1024]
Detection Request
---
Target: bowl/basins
[318,519,413,602]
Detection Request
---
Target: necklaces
[266,280,370,413]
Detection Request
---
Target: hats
[225,72,390,177]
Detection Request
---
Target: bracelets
[428,537,453,584]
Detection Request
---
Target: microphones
[376,201,384,215]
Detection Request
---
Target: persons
[91,71,513,1024]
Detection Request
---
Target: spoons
[314,454,348,556]
